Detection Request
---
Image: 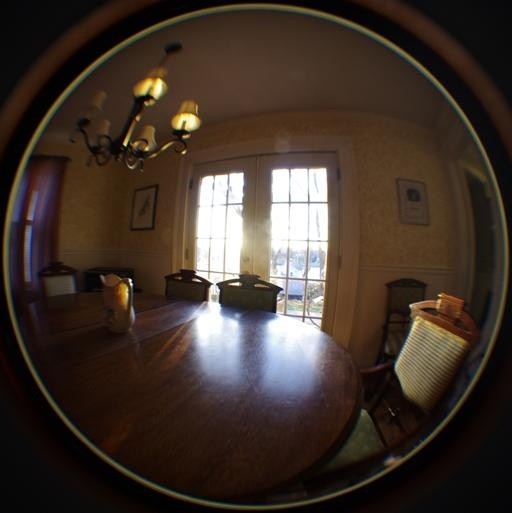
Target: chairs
[303,292,479,498]
[375,276,428,364]
[83,266,144,294]
[163,268,213,302]
[216,274,283,313]
[36,261,80,298]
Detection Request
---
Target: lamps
[68,39,202,175]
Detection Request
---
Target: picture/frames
[128,184,159,230]
[394,177,431,225]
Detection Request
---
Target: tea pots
[98,272,136,332]
